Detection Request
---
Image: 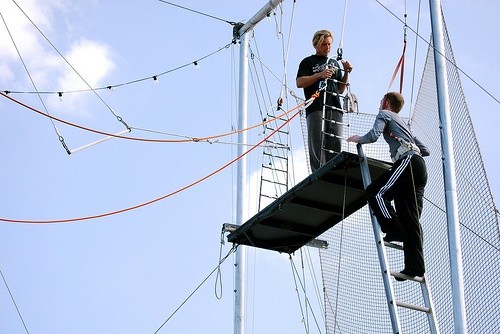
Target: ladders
[356,142,440,334]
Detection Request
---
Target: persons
[346,92,430,281]
[296,29,353,173]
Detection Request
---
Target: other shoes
[395,269,425,283]
[378,235,404,246]
